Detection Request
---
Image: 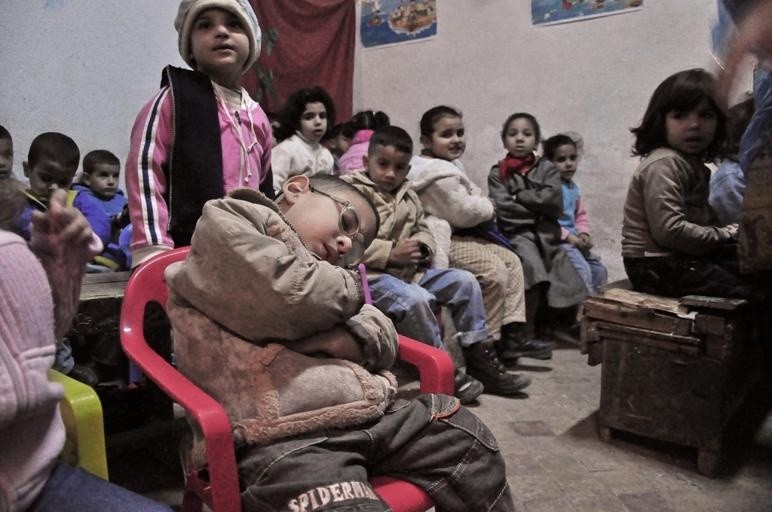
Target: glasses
[310,185,364,268]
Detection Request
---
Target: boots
[462,336,529,394]
[534,308,553,337]
[455,371,484,402]
[558,302,581,338]
[502,323,556,359]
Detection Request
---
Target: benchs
[580,273,768,477]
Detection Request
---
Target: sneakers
[68,364,98,387]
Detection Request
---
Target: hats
[175,0,262,75]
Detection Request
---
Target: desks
[71,270,137,301]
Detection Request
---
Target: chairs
[45,368,111,482]
[118,243,461,512]
